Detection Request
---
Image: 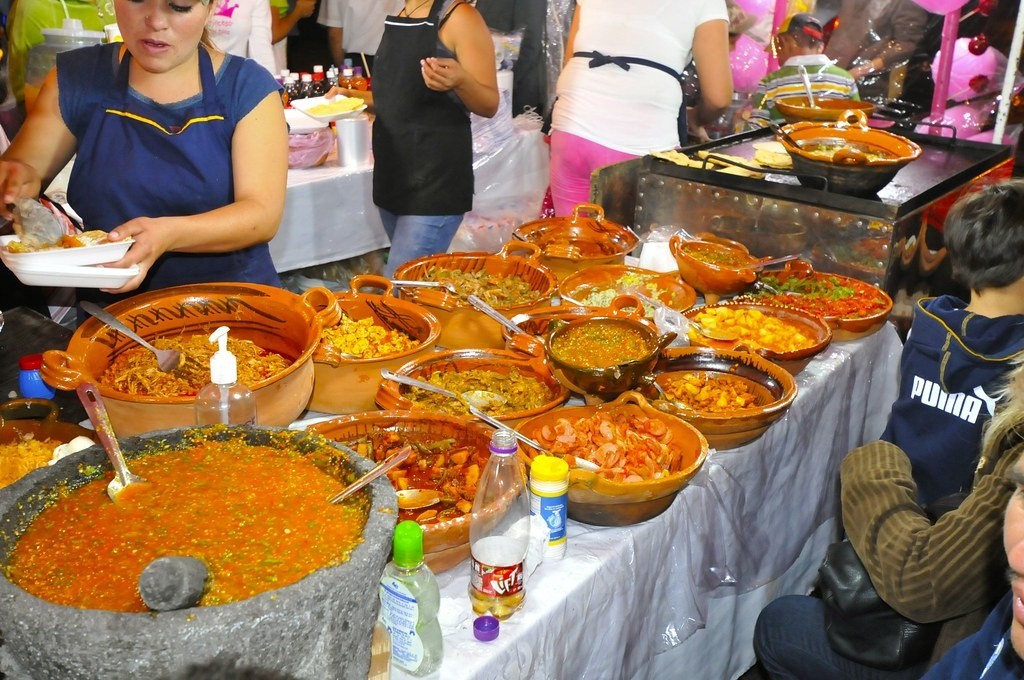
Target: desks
[264,164,400,275]
[0,229,906,680]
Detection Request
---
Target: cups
[105,23,122,45]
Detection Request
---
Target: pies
[309,97,364,115]
[652,141,793,181]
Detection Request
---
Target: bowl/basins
[0,201,893,572]
[775,96,878,123]
[778,107,922,198]
[0,424,397,680]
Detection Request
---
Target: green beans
[335,434,460,516]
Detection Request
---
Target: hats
[775,13,824,40]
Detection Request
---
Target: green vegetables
[758,276,854,301]
[422,265,540,306]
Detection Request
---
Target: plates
[290,94,367,120]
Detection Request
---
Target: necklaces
[405,0,429,17]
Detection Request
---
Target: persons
[745,14,861,131]
[0,0,577,131]
[325,0,499,298]
[0,0,291,330]
[823,0,1024,117]
[550,0,749,218]
[753,178,1024,680]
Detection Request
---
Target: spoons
[468,404,600,472]
[77,384,154,509]
[391,280,456,292]
[395,489,444,511]
[380,369,507,410]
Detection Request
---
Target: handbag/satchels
[818,538,944,669]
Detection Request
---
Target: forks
[81,301,204,382]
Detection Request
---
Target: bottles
[275,58,368,109]
[376,520,444,677]
[467,431,530,619]
[531,454,567,561]
[20,355,56,402]
